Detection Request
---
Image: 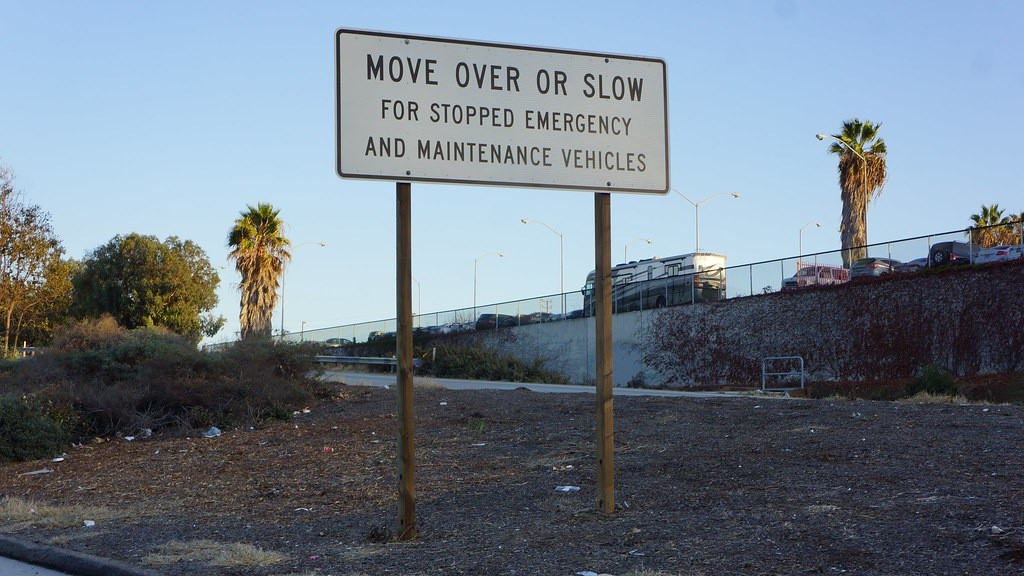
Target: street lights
[695,192,739,253]
[302,322,307,340]
[816,134,868,258]
[521,219,564,314]
[282,242,326,333]
[474,253,505,321]
[800,221,821,269]
[625,239,653,263]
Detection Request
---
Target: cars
[851,257,901,277]
[413,309,583,334]
[319,338,352,348]
[368,331,397,342]
[894,258,927,273]
[974,245,1024,263]
[782,266,850,289]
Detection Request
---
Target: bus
[581,252,726,317]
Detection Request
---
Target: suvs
[926,241,985,266]
